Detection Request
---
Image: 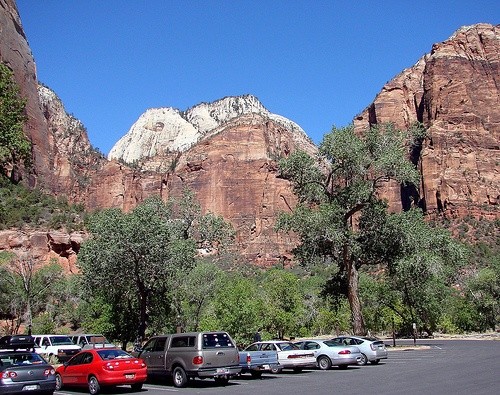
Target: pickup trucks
[239,351,278,377]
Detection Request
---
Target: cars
[243,339,317,373]
[332,336,388,366]
[283,339,362,369]
[0,334,36,352]
[0,351,57,395]
[55,348,147,394]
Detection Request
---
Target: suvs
[130,331,241,388]
[69,334,117,349]
[31,334,81,363]
